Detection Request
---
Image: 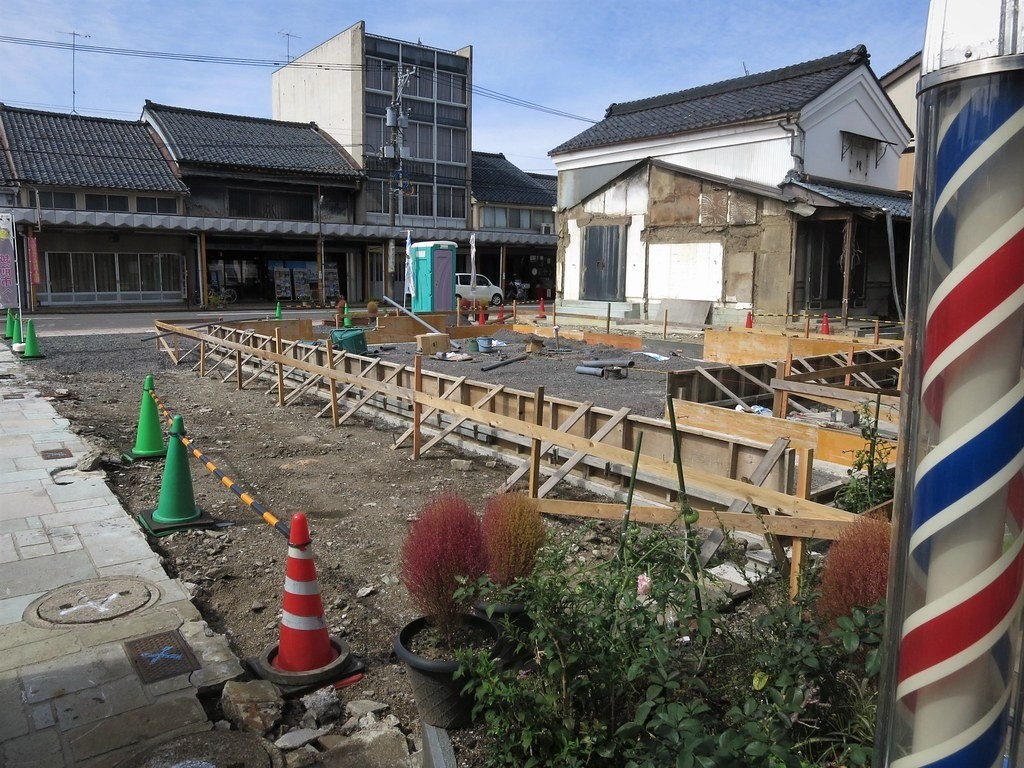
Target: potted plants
[472,478,546,662]
[209,296,221,310]
[392,488,505,731]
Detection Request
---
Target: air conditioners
[539,222,554,235]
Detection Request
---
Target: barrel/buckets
[468,338,478,353]
[476,336,493,353]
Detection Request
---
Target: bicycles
[193,279,239,311]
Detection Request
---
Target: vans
[454,273,504,308]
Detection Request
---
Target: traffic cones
[496,303,506,325]
[137,412,215,537]
[4,306,16,340]
[10,313,25,349]
[820,312,831,334]
[241,510,368,700]
[19,321,45,364]
[116,374,169,461]
[478,306,493,325]
[745,311,753,329]
[274,302,283,320]
[340,303,354,328]
[536,297,548,319]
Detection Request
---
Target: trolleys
[314,326,392,360]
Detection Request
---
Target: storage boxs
[416,333,451,355]
